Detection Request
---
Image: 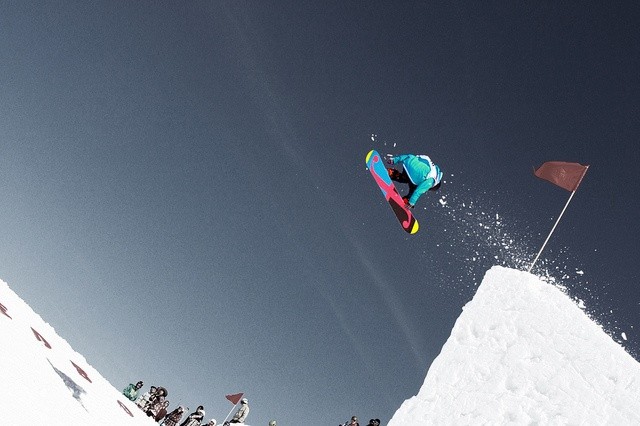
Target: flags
[534,161,586,190]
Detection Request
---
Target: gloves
[404,202,413,210]
[384,155,394,164]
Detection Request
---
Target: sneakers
[402,195,409,205]
[387,167,400,180]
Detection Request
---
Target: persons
[384,153,444,206]
[187,410,206,425]
[339,416,360,426]
[179,406,204,426]
[156,401,169,420]
[368,418,374,424]
[375,420,382,425]
[122,381,143,401]
[135,386,156,408]
[144,387,169,418]
[225,398,249,422]
[207,419,216,426]
[160,406,184,426]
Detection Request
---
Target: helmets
[136,381,143,388]
[269,420,276,425]
[151,385,157,388]
[351,416,357,422]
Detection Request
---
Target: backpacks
[155,407,167,422]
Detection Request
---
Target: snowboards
[365,150,419,234]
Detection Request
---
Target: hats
[157,386,168,396]
[209,419,217,426]
[199,410,205,415]
[179,405,184,411]
[242,398,248,404]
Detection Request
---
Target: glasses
[371,421,374,422]
[165,401,168,404]
